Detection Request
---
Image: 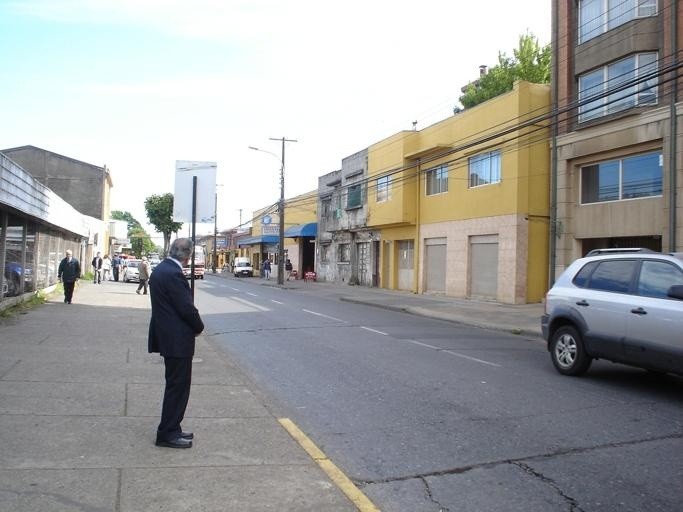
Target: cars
[123,260,149,282]
[0,247,63,297]
[149,259,161,270]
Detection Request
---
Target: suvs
[541,248,682,378]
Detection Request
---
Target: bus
[179,244,204,280]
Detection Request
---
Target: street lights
[247,146,286,285]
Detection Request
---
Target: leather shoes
[179,432,193,439]
[157,437,192,448]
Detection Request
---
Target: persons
[136,256,149,294]
[93,251,124,284]
[285,259,292,281]
[148,238,204,448]
[58,249,81,304]
[263,259,271,280]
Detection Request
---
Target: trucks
[232,256,254,277]
